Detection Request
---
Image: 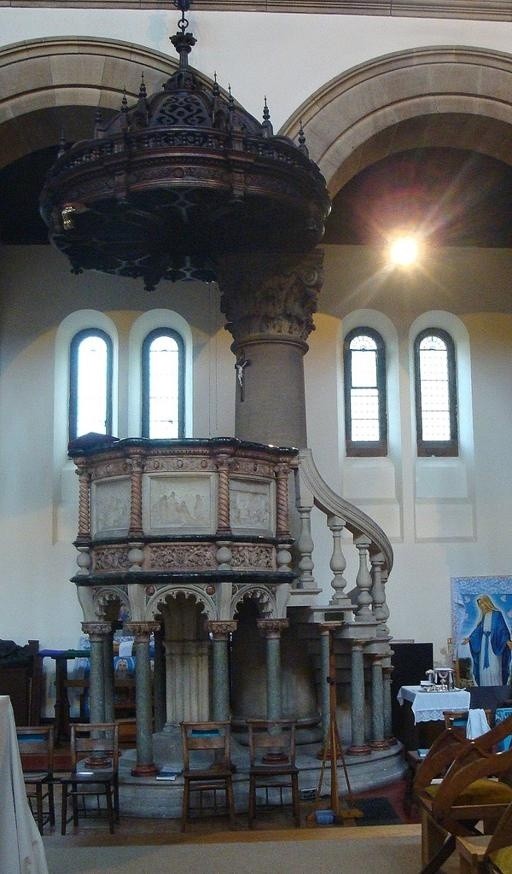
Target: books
[78,767,95,774]
[417,748,429,757]
[156,767,182,780]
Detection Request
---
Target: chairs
[56,721,122,836]
[407,707,512,874]
[176,718,239,832]
[245,717,300,829]
[16,725,56,834]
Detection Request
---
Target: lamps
[33,1,331,292]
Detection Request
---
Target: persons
[460,595,512,688]
[115,659,132,678]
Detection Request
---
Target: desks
[398,685,472,758]
